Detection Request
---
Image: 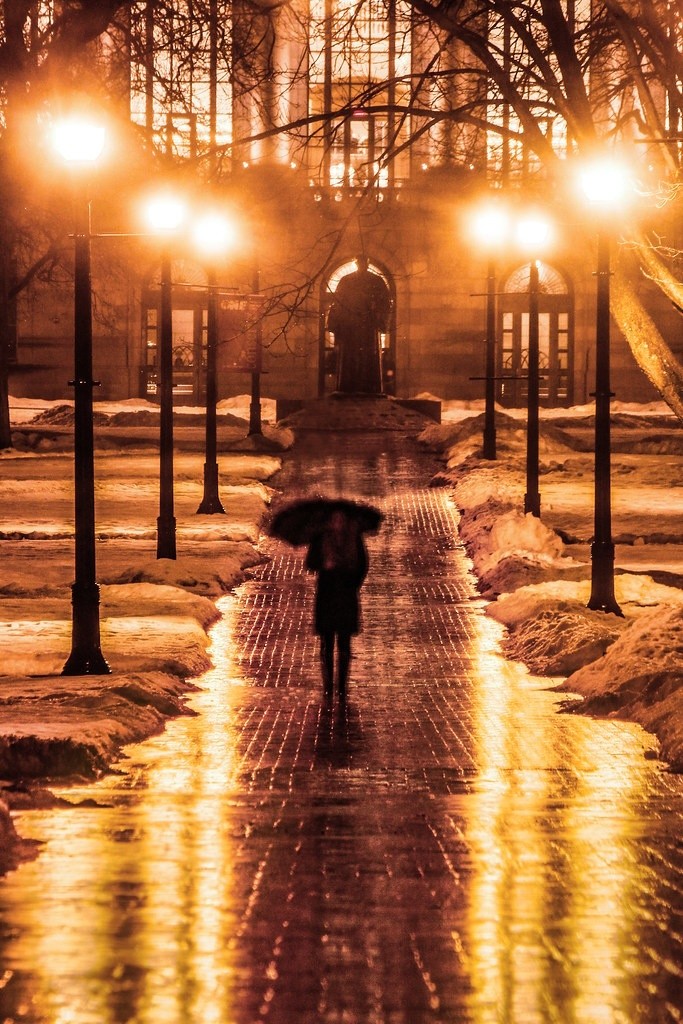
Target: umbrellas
[264,498,386,570]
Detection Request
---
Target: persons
[306,512,369,716]
[328,252,392,397]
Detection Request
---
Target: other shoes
[339,692,346,698]
[323,690,333,699]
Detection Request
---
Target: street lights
[147,192,185,564]
[564,152,637,613]
[473,214,508,462]
[59,132,111,678]
[193,213,228,514]
[516,212,552,522]
